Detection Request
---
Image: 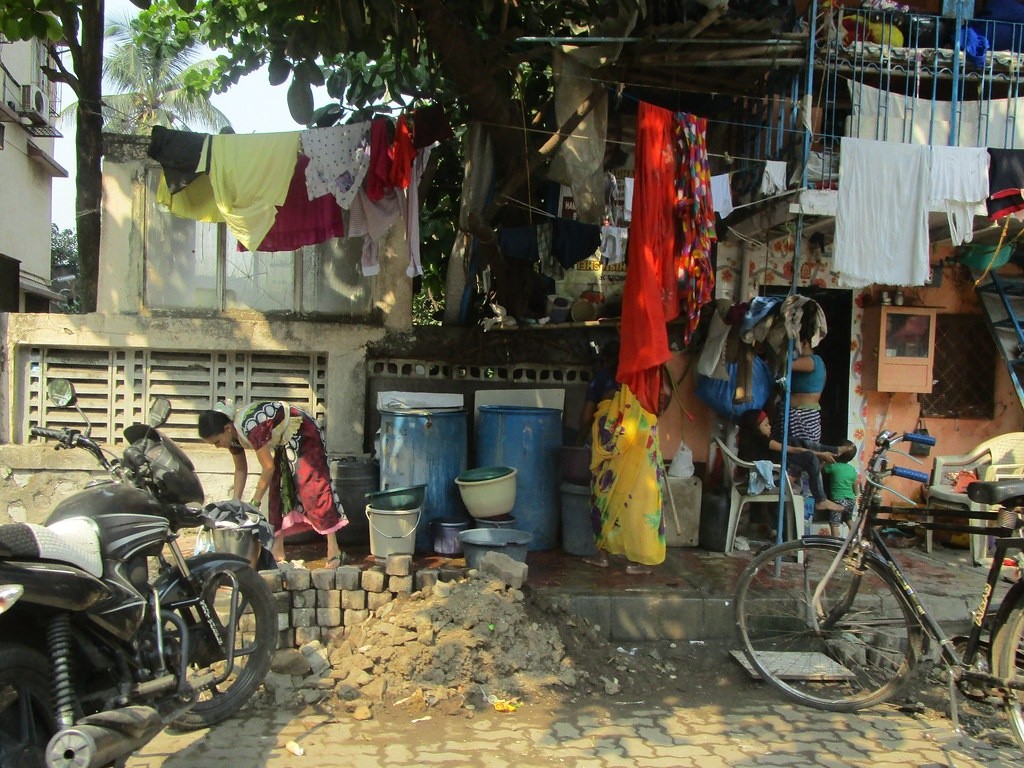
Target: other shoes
[626,565,654,574]
[582,556,609,568]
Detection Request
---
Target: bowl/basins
[457,528,532,570]
[365,483,427,510]
[455,467,518,516]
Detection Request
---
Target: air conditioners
[21,84,50,124]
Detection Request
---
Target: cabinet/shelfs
[865,305,937,393]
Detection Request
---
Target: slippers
[324,548,342,570]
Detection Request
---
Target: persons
[739,324,858,538]
[199,400,349,569]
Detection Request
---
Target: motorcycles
[0,379,279,768]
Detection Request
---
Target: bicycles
[732,430,1024,753]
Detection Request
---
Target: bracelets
[250,499,261,507]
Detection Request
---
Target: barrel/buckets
[474,516,516,529]
[475,405,564,551]
[330,458,379,546]
[428,518,469,555]
[380,412,468,551]
[560,476,598,556]
[365,504,421,558]
[211,529,261,572]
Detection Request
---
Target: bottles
[894,291,903,305]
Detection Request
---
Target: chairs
[714,436,807,564]
[926,432,1024,565]
